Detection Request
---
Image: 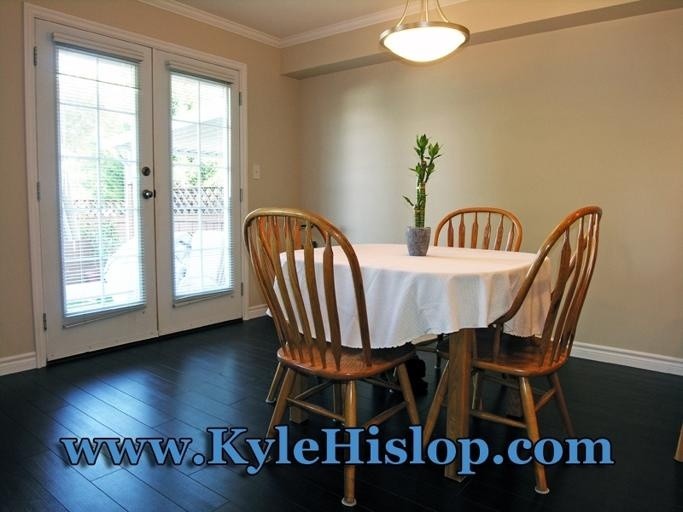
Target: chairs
[239,206,422,508]
[426,207,522,368]
[415,204,603,494]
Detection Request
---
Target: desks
[258,240,552,483]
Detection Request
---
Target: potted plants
[402,132,439,256]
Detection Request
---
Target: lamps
[376,0,471,68]
